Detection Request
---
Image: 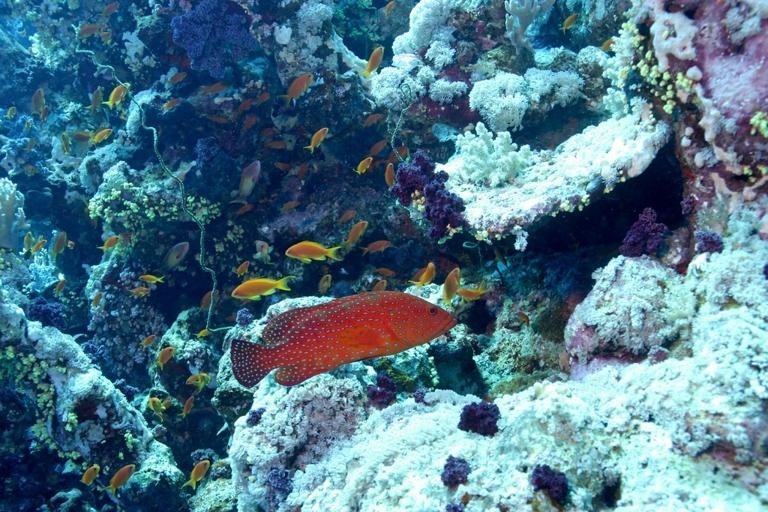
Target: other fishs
[0,0,493,426]
